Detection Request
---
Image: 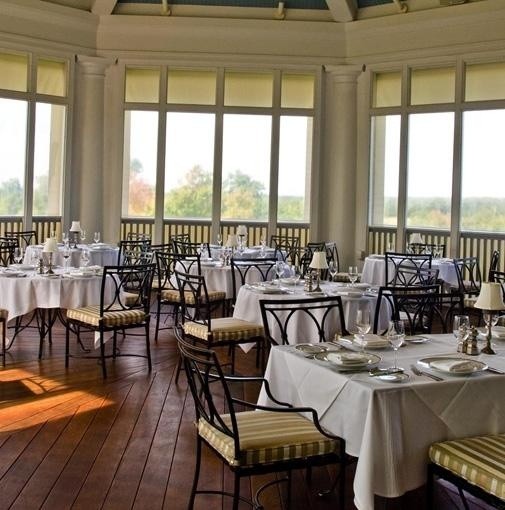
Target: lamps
[407,232,422,254]
[307,250,330,293]
[473,282,505,353]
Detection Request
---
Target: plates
[417,356,490,377]
[313,350,382,373]
[291,342,327,356]
[406,335,430,344]
[375,372,411,383]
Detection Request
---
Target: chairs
[0,236,19,266]
[169,232,192,244]
[2,230,39,265]
[484,252,505,302]
[393,293,464,335]
[392,263,439,295]
[236,223,249,253]
[259,296,347,345]
[155,251,227,346]
[0,308,8,364]
[124,232,152,242]
[454,255,487,300]
[372,285,438,335]
[65,264,154,378]
[270,234,301,269]
[69,220,82,248]
[171,240,212,263]
[174,269,265,384]
[42,237,58,275]
[230,257,278,307]
[171,327,346,510]
[403,242,447,260]
[301,242,337,282]
[165,244,295,288]
[272,243,309,278]
[225,234,239,263]
[428,434,505,510]
[115,240,153,319]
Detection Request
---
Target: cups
[29,246,40,278]
[61,232,69,250]
[355,309,371,354]
[439,246,443,260]
[260,234,267,249]
[94,232,101,245]
[276,260,285,289]
[61,246,72,275]
[199,242,208,263]
[289,264,301,293]
[80,249,90,273]
[216,233,223,249]
[80,230,86,245]
[50,230,57,240]
[452,315,471,358]
[328,261,338,287]
[348,266,358,293]
[433,246,438,260]
[14,247,23,271]
[238,244,246,259]
[222,249,229,268]
[482,314,499,339]
[386,321,405,372]
